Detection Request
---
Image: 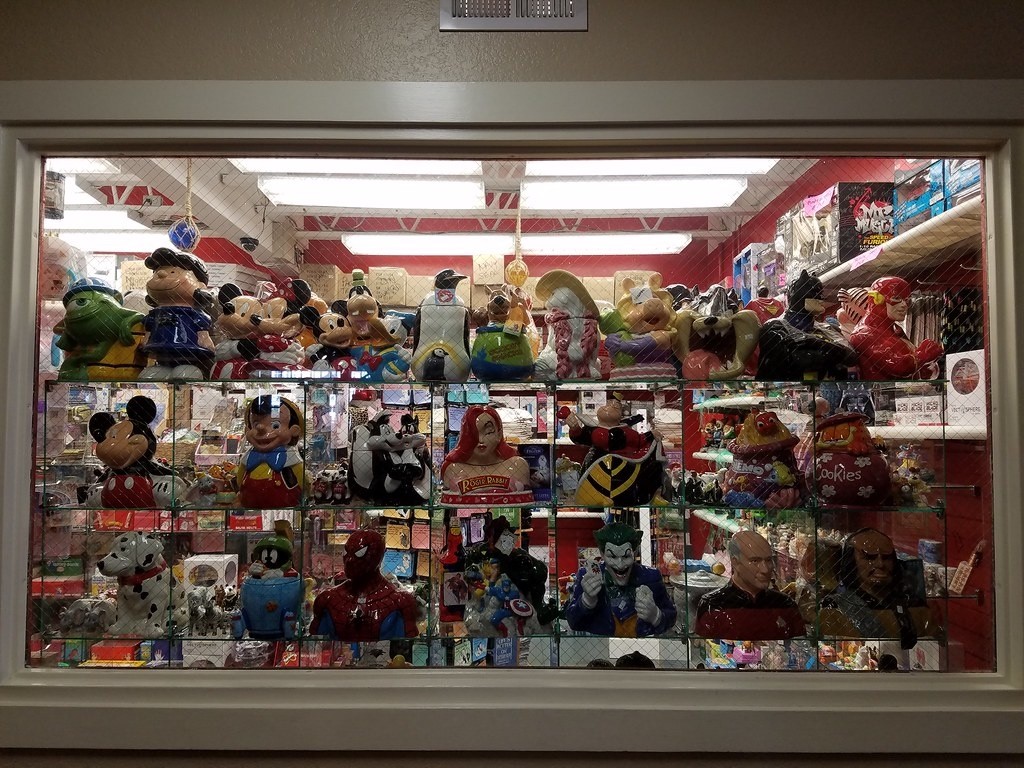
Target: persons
[307,528,420,641]
[691,526,943,641]
[744,286,785,376]
[850,276,944,383]
[754,269,860,382]
[442,404,556,636]
[563,397,677,639]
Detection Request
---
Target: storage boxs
[733,159,983,306]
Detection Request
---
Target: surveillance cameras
[239,238,259,253]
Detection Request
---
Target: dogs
[95,529,191,639]
